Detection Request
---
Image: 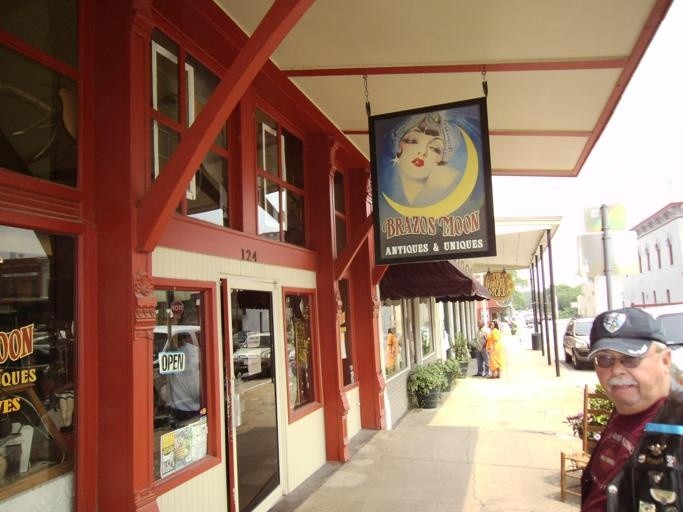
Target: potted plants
[407,329,468,408]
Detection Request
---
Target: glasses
[594,353,642,368]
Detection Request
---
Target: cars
[645,304,683,389]
[0,329,55,366]
[153,323,202,389]
[508,308,553,325]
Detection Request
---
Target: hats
[587,308,666,359]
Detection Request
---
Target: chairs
[562,384,627,504]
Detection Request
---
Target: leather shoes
[474,374,499,378]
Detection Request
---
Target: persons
[161,323,200,424]
[471,320,490,377]
[388,111,459,205]
[485,320,504,378]
[579,307,682,511]
[385,326,401,366]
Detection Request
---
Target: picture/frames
[367,95,497,267]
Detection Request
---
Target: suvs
[563,314,597,371]
[233,331,273,382]
